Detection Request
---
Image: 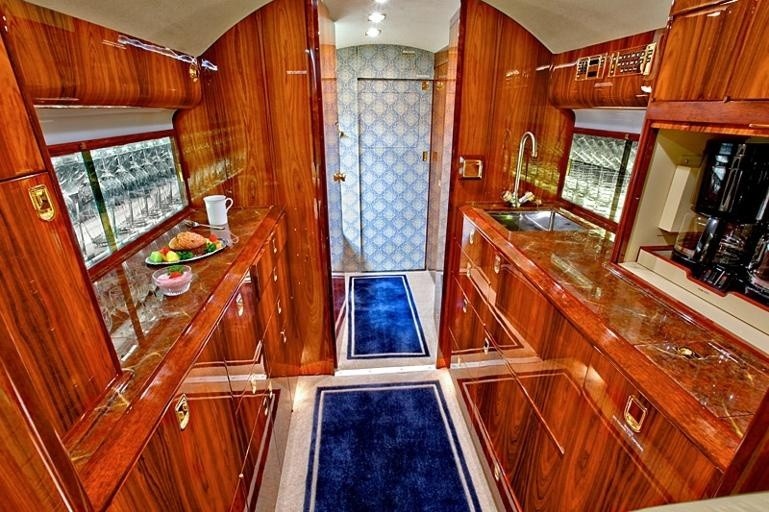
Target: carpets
[301,379,479,512]
[346,272,431,359]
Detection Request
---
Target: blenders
[670,134,769,307]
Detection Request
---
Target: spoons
[184,218,225,231]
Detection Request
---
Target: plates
[145,232,226,266]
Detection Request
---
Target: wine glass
[94,219,192,347]
[50,137,183,260]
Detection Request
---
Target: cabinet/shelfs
[648,4,769,132]
[505,320,721,512]
[443,210,498,387]
[247,209,305,410]
[108,323,252,511]
[216,271,277,466]
[46,129,197,283]
[0,173,124,442]
[466,263,553,489]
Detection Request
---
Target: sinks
[526,202,598,232]
[482,206,544,233]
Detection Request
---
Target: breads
[168,232,206,250]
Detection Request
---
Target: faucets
[501,131,538,208]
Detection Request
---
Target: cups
[201,193,233,228]
[564,132,640,216]
